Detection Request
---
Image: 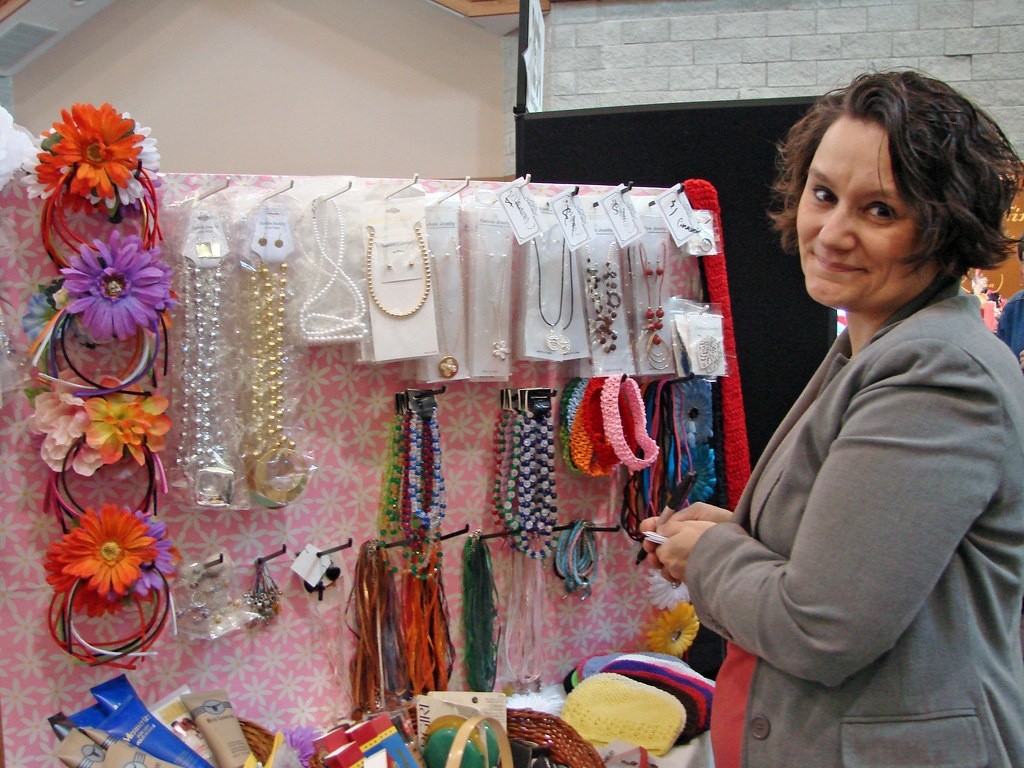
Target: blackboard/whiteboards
[515,94,838,683]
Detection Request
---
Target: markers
[635,471,698,566]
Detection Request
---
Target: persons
[995,233,1024,370]
[639,66,1024,768]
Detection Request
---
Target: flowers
[35,104,162,215]
[45,504,177,617]
[27,377,168,473]
[21,229,175,344]
[644,570,700,657]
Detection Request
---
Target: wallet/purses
[560,649,717,757]
